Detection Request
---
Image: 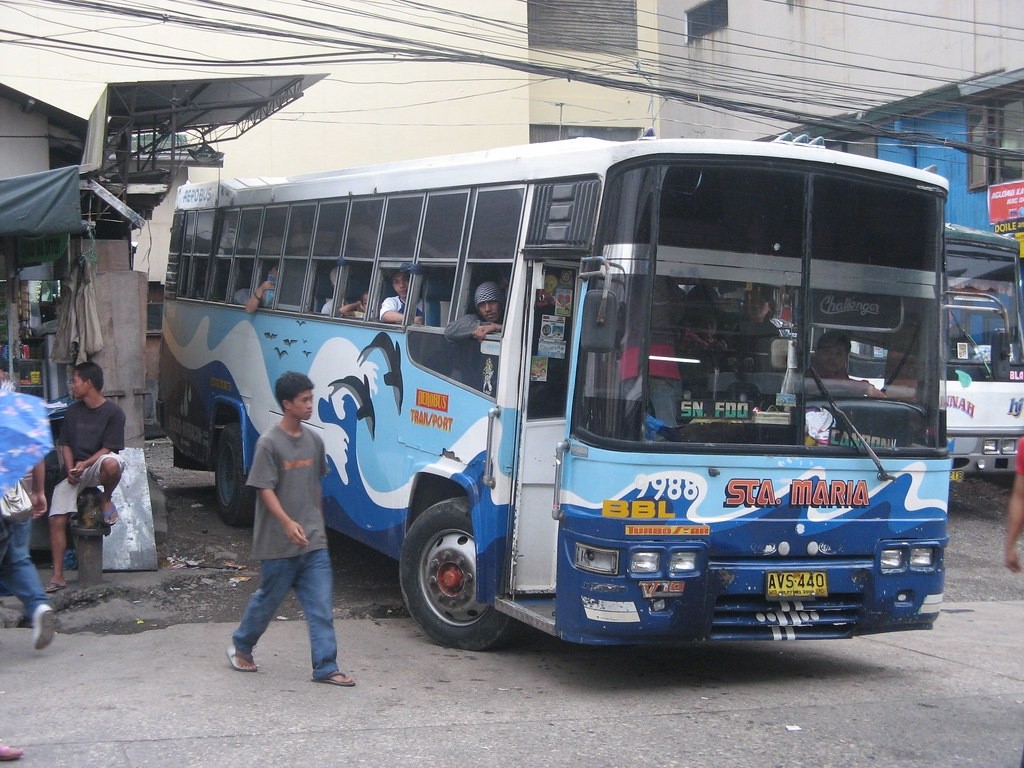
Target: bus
[156,137,1010,651]
[943,221,1024,480]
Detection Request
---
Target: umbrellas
[0,389,54,499]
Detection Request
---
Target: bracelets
[254,293,262,300]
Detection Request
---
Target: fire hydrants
[72,487,111,587]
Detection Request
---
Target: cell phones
[70,469,78,472]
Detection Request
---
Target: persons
[340,291,368,318]
[322,267,337,314]
[794,333,886,398]
[225,372,355,687]
[1005,436,1024,572]
[619,280,782,439]
[246,262,278,313]
[379,269,424,325]
[0,459,55,648]
[42,361,126,593]
[443,281,505,342]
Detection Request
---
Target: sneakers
[0,744,23,760]
[33,604,54,650]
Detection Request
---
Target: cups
[21,345,30,359]
[71,469,80,483]
[2,345,9,358]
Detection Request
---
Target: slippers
[44,583,65,592]
[311,672,355,686]
[225,647,258,671]
[102,504,119,525]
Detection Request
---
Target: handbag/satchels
[0,480,33,522]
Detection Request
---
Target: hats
[474,281,505,311]
[382,266,401,281]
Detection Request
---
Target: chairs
[213,271,447,326]
